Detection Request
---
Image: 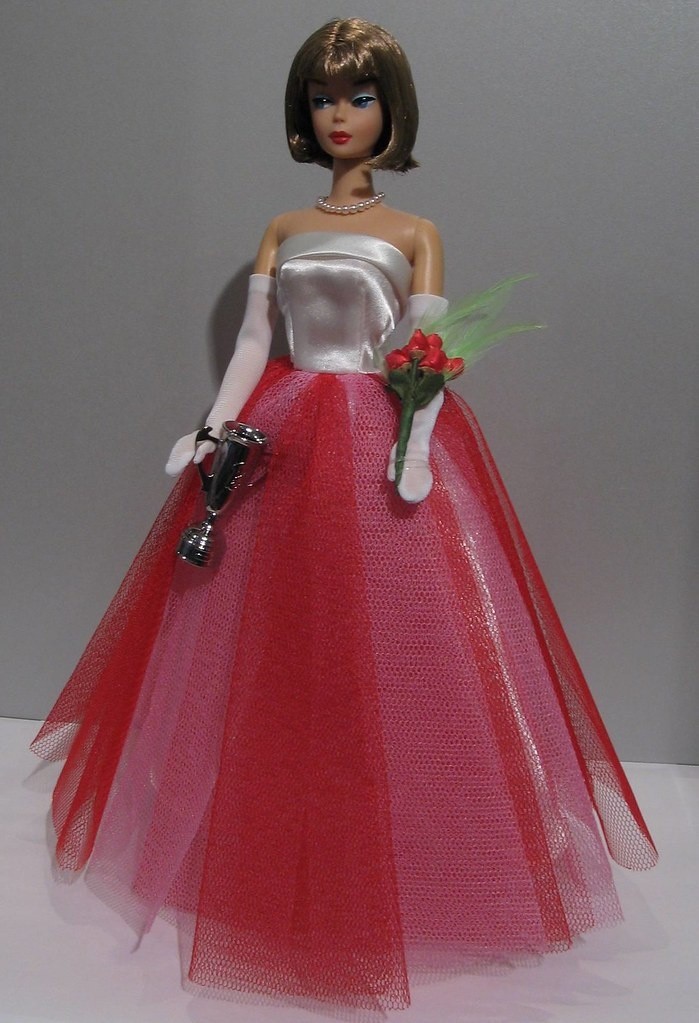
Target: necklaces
[316,192,386,215]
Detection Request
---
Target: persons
[31,18,656,1020]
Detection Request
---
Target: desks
[0,716,699,1023]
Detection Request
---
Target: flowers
[381,326,467,480]
